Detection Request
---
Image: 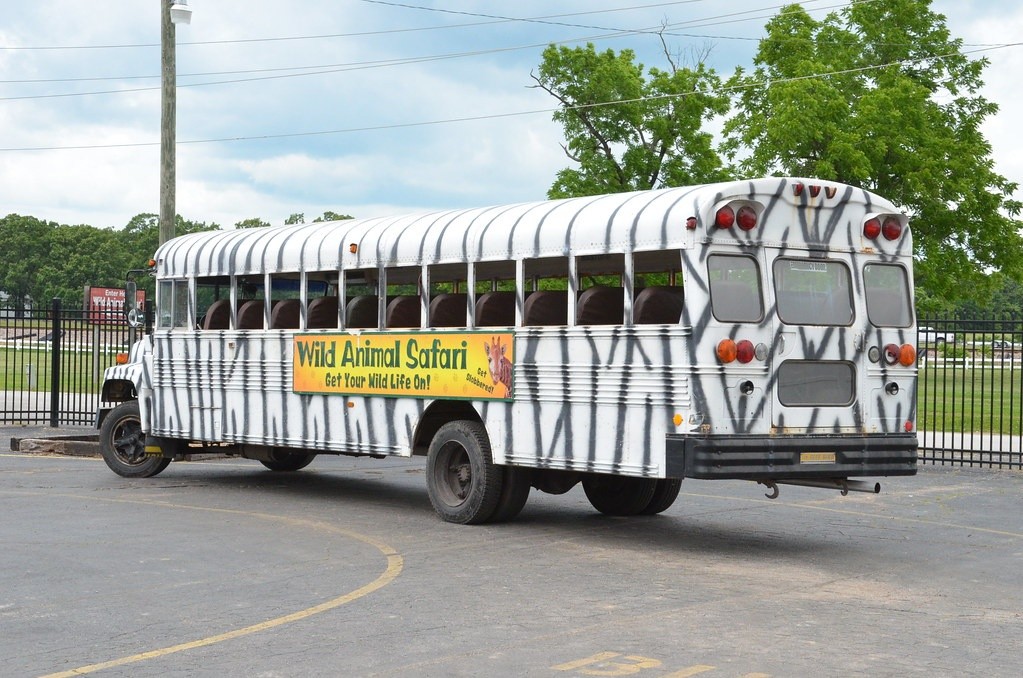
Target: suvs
[918,326,955,346]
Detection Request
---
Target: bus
[93,176,922,533]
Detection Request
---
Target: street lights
[159,0,191,255]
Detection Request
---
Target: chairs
[203,282,830,329]
